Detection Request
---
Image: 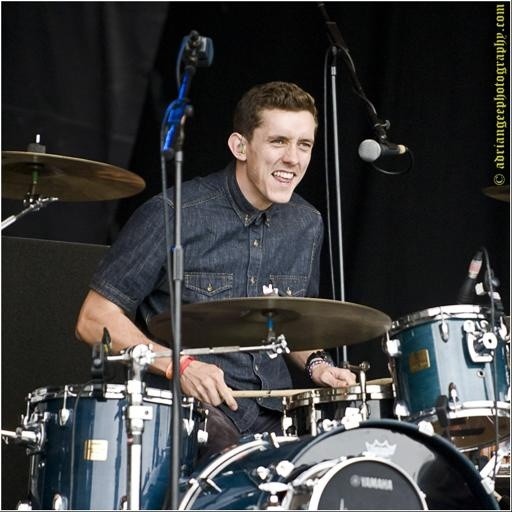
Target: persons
[74,81,359,453]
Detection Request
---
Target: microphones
[182,30,214,67]
[358,138,411,163]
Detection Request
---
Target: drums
[284,385,395,436]
[386,305,512,451]
[180,419,503,511]
[27,383,202,512]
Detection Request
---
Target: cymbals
[2,149,146,203]
[149,296,395,352]
[482,185,510,202]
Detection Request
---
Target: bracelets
[307,360,332,377]
[166,354,188,379]
[305,350,333,374]
[180,356,193,378]
